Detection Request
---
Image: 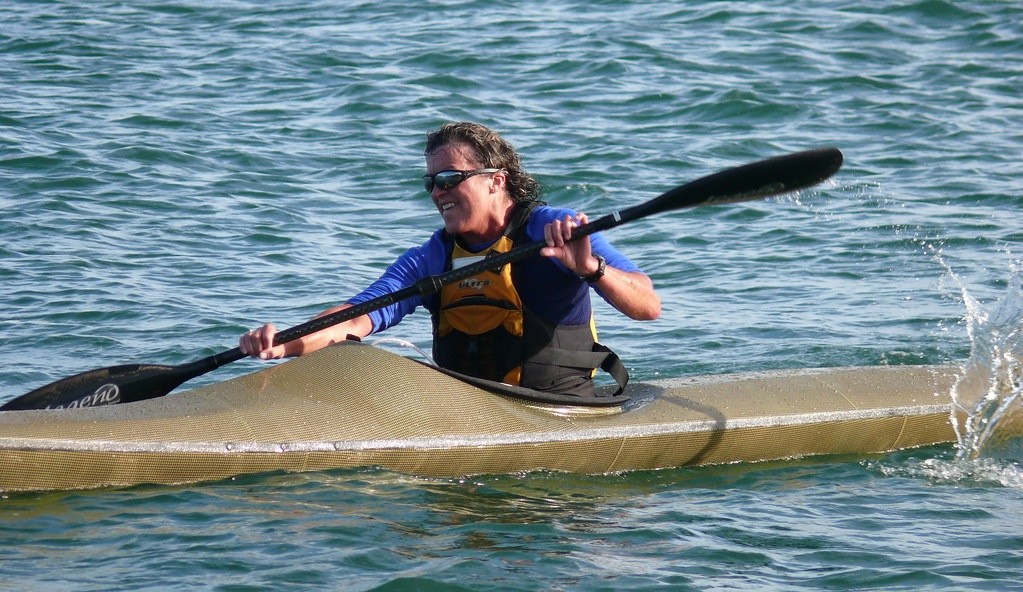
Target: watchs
[579,254,606,284]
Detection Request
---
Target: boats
[0,332,1023,495]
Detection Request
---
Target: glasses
[422,168,507,193]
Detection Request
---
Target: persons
[240,122,661,397]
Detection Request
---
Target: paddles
[0,146,843,411]
